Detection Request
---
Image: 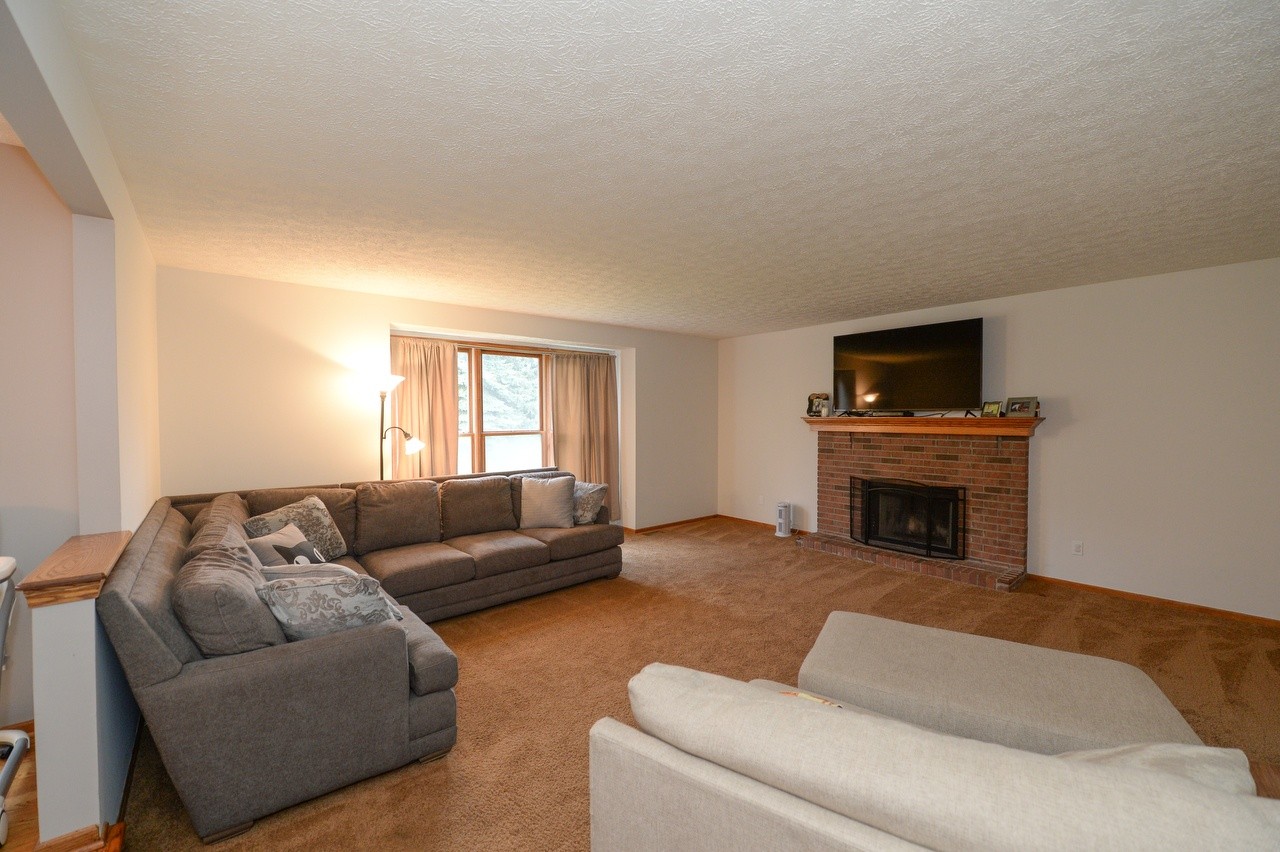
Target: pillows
[171,471,608,658]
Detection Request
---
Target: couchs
[96,468,624,844]
[589,662,1280,852]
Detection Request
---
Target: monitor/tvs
[831,317,985,416]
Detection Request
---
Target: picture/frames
[981,402,1002,418]
[1005,397,1037,417]
[807,394,829,417]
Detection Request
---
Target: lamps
[372,375,426,480]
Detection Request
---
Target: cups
[820,400,829,417]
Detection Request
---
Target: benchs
[798,611,1205,755]
